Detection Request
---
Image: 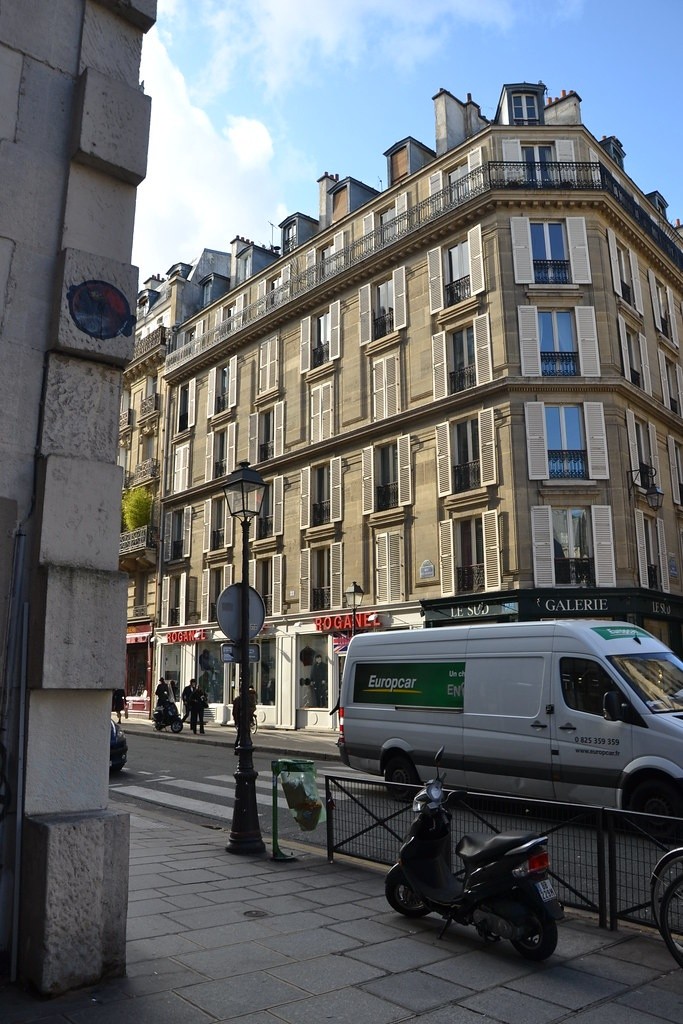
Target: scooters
[153,692,183,734]
[386,744,567,962]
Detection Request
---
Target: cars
[110,718,128,776]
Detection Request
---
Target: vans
[335,620,683,843]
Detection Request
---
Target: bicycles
[650,847,683,969]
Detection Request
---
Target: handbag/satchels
[202,700,208,708]
[125,708,128,719]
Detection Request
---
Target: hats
[159,678,164,681]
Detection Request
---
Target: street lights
[222,460,271,852]
[344,582,364,636]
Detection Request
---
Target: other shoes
[193,729,196,734]
[117,721,121,724]
[200,730,204,734]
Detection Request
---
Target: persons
[182,679,208,735]
[329,695,340,745]
[233,686,258,749]
[155,677,178,714]
[113,689,127,724]
[311,655,328,707]
